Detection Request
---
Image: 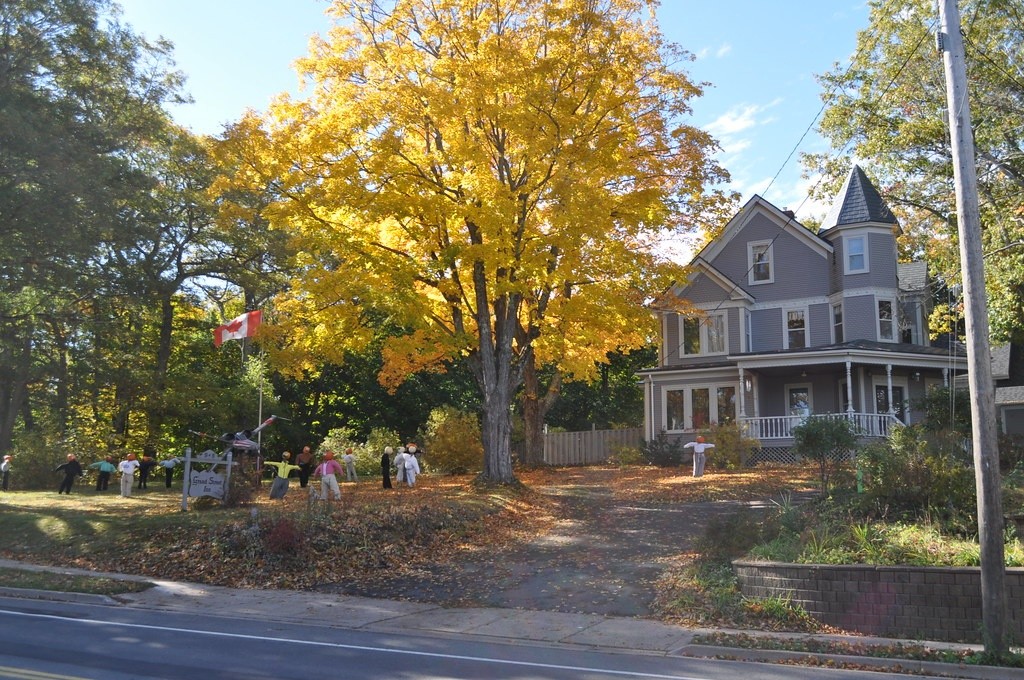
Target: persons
[54,453,180,500]
[1,455,12,492]
[262,443,419,502]
[683,436,715,478]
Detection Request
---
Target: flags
[215,310,262,348]
